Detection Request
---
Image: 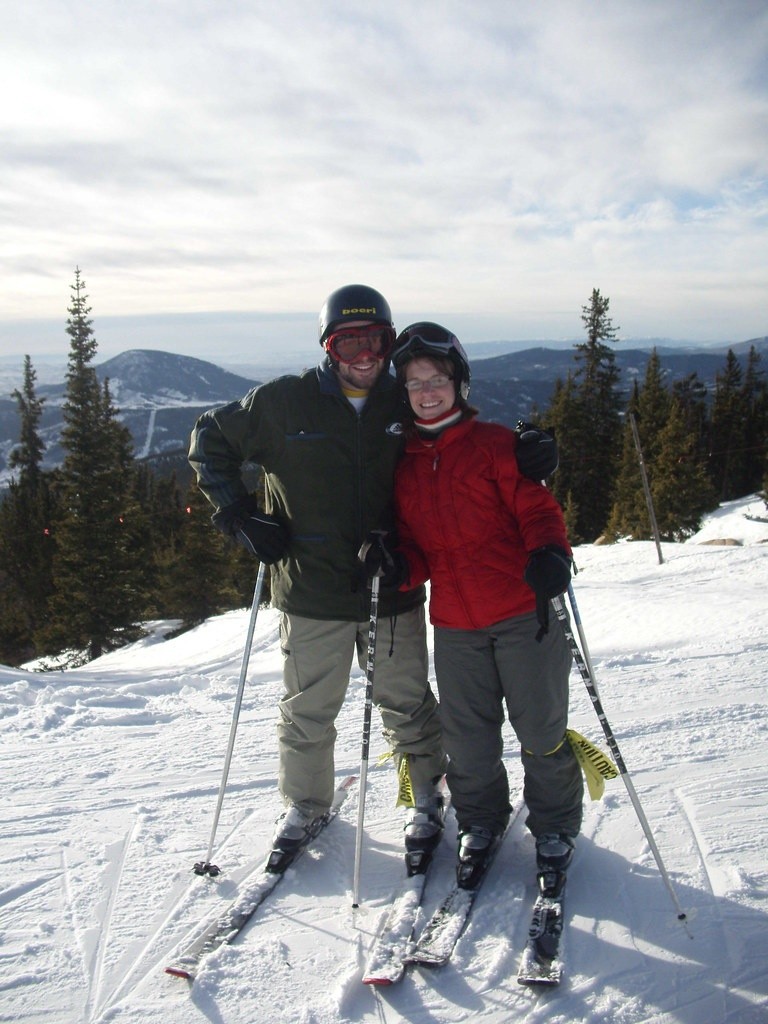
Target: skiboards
[401,789,525,969]
[517,863,566,987]
[363,787,454,985]
[164,775,353,981]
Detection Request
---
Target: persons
[191,280,559,856]
[349,320,587,899]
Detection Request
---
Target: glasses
[404,374,453,391]
[391,322,470,368]
[322,325,396,365]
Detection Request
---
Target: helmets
[320,284,392,345]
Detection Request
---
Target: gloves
[514,423,560,482]
[524,548,571,600]
[241,513,287,567]
[365,548,402,584]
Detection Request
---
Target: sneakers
[459,820,501,864]
[271,805,322,850]
[403,803,442,852]
[535,833,577,872]
